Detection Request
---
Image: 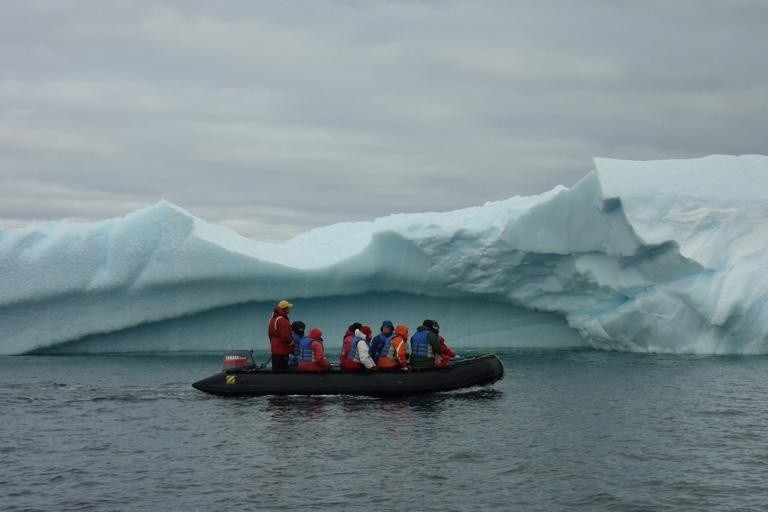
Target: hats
[310,329,321,339]
[278,301,293,309]
[349,320,440,342]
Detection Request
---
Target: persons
[298,328,332,372]
[340,319,461,371]
[287,320,306,369]
[268,300,294,370]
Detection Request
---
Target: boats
[192,355,504,398]
